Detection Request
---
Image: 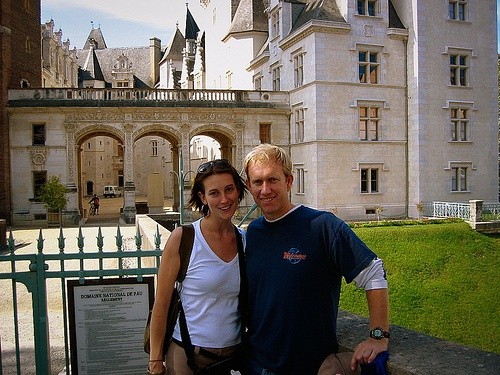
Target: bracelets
[147,365,166,375]
[148,359,165,363]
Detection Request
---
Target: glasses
[198,159,231,173]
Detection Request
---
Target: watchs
[370,327,390,340]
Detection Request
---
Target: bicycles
[89,202,95,215]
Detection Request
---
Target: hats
[317,352,361,375]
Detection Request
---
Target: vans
[103,185,121,198]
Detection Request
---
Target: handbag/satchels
[144,225,195,362]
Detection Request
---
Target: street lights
[170,149,198,227]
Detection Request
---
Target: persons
[147,158,247,375]
[242,142,390,375]
[88,194,100,215]
